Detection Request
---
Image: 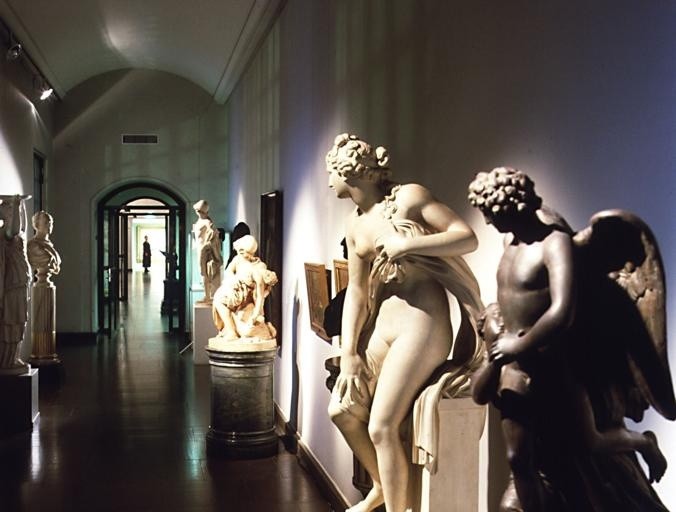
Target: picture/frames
[333,259,348,346]
[303,261,332,342]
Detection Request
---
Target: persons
[25,210,62,356]
[0,194,31,369]
[326,133,485,512]
[143,236,151,272]
[190,199,223,304]
[213,234,278,341]
[467,167,676,512]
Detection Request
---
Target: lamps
[35,75,52,101]
[5,31,22,60]
